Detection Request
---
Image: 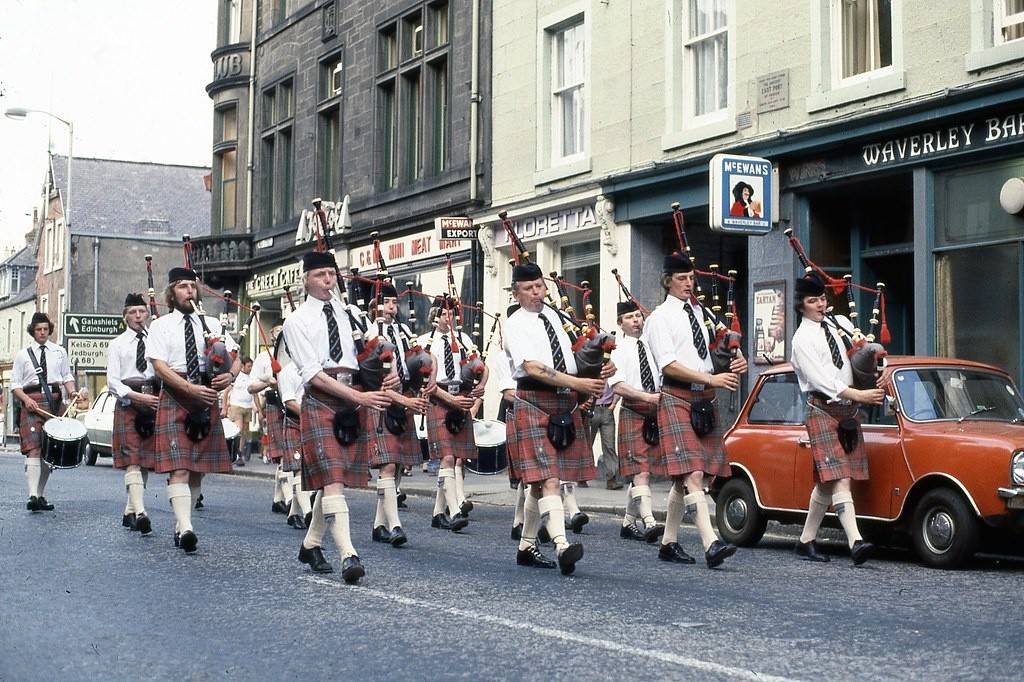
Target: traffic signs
[64,314,127,338]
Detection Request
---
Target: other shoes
[262,455,269,465]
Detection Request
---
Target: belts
[26,379,862,408]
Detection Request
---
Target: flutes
[139,254,175,334]
[183,235,262,403]
[445,249,500,424]
[672,200,743,413]
[787,227,903,416]
[609,266,652,321]
[351,230,450,431]
[310,196,397,433]
[280,285,295,309]
[497,209,616,416]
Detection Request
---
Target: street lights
[5,108,74,314]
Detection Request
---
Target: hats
[168,266,197,283]
[795,272,826,298]
[430,294,455,309]
[302,251,337,273]
[616,301,642,317]
[31,313,49,323]
[512,261,543,283]
[662,251,695,276]
[371,280,398,301]
[123,294,145,306]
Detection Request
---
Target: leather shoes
[29,492,881,584]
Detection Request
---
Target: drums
[39,415,88,470]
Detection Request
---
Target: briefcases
[220,417,240,466]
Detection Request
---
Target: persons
[501,253,748,575]
[792,273,891,566]
[249,252,490,585]
[105,267,254,556]
[0,312,93,510]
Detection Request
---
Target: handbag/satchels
[333,391,861,461]
[133,410,156,439]
[184,411,213,444]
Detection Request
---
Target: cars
[77,384,121,466]
[708,352,1023,569]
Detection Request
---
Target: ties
[321,304,344,364]
[386,326,406,380]
[134,333,147,374]
[682,303,708,361]
[38,345,49,386]
[820,321,845,369]
[181,315,202,384]
[441,335,455,383]
[537,314,568,379]
[636,341,655,392]
